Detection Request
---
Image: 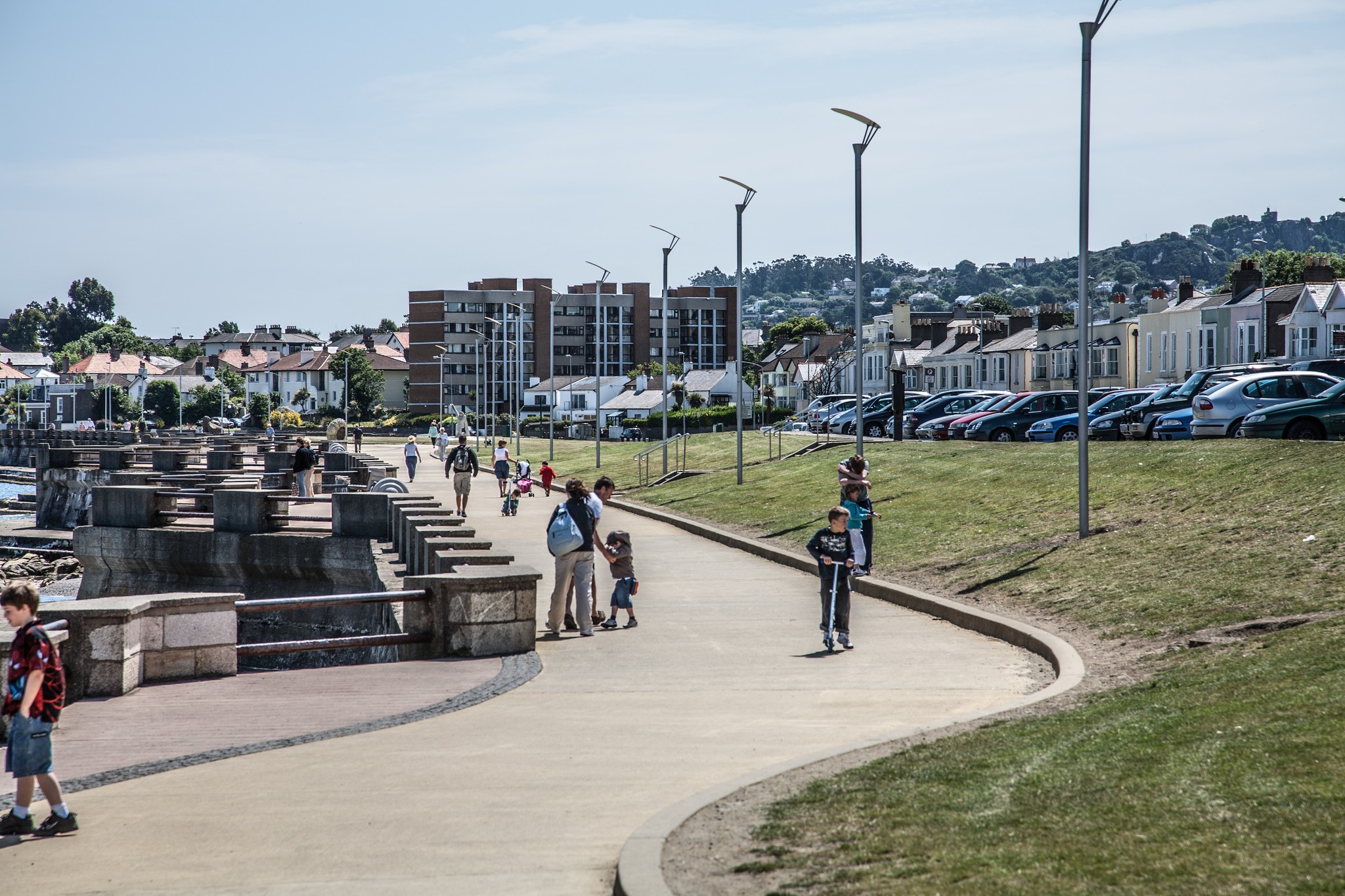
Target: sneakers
[591,611,605,624]
[580,630,595,636]
[837,634,854,648]
[622,619,638,628]
[33,810,78,837]
[457,510,466,517]
[601,619,617,628]
[822,633,835,646]
[0,807,34,835]
[564,613,577,629]
[545,618,560,634]
[851,568,867,577]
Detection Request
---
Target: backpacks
[452,445,471,471]
[315,455,319,464]
[548,503,584,558]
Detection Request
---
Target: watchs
[124,419,131,431]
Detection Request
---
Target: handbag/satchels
[272,434,274,437]
[291,474,299,496]
[435,440,441,446]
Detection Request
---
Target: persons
[44,421,55,437]
[404,436,421,483]
[137,421,140,427]
[135,427,140,433]
[838,454,876,576]
[501,489,521,516]
[292,438,312,505]
[48,425,52,430]
[302,438,316,504]
[600,530,639,630]
[444,434,479,518]
[352,425,363,453]
[428,422,439,448]
[545,479,598,637]
[194,420,203,433]
[805,505,856,648]
[436,427,450,462]
[491,437,518,498]
[86,418,95,431]
[840,483,883,577]
[430,420,439,434]
[539,460,556,497]
[79,422,86,430]
[1,581,83,841]
[265,424,275,441]
[139,419,146,437]
[563,474,616,629]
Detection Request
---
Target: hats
[603,531,618,545]
[408,435,416,442]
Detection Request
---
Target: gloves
[429,436,430,438]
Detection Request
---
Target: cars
[806,358,1345,441]
[229,418,244,428]
[145,420,158,429]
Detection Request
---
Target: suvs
[211,417,234,429]
[620,428,643,443]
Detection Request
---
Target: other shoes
[512,513,516,516]
[295,501,315,505]
[441,458,446,461]
[433,446,435,448]
[359,450,360,453]
[501,493,505,498]
[545,487,549,497]
[410,478,413,483]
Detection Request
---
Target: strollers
[513,459,535,497]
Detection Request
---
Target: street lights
[678,351,685,435]
[880,320,890,393]
[433,355,453,436]
[565,354,572,437]
[340,349,352,439]
[434,344,447,431]
[106,362,112,430]
[719,176,758,487]
[41,373,49,429]
[541,285,564,461]
[585,261,610,469]
[830,107,883,462]
[649,225,680,476]
[468,328,483,450]
[735,359,764,430]
[18,378,22,429]
[268,358,274,425]
[478,334,492,448]
[139,365,146,421]
[484,316,502,456]
[264,371,282,431]
[71,369,77,424]
[1252,238,1267,362]
[972,303,985,389]
[504,339,516,443]
[506,301,527,455]
[803,338,810,405]
